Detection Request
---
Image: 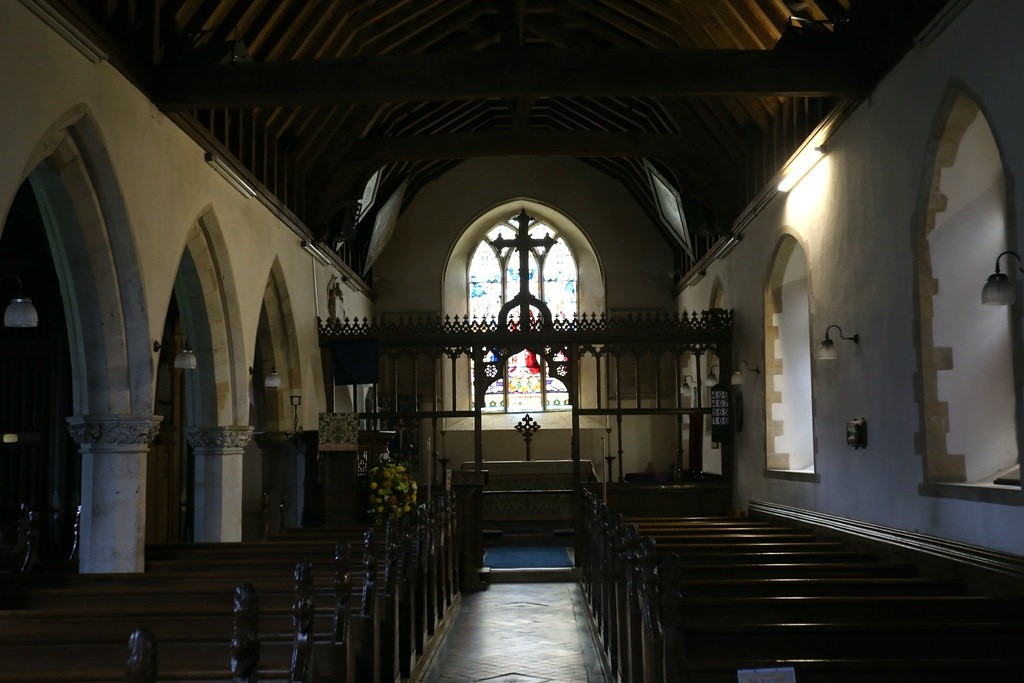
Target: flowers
[369,451,421,531]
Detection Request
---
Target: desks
[459,460,600,535]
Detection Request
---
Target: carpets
[484,545,575,567]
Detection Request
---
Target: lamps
[817,325,860,359]
[20,0,109,64]
[730,360,759,385]
[705,364,719,387]
[153,331,198,369]
[302,240,332,266]
[680,375,697,397]
[342,275,363,292]
[981,250,1024,306]
[248,359,282,387]
[777,145,826,192]
[205,152,258,200]
[0,272,39,327]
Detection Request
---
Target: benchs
[581,484,1024,683]
[1,489,459,683]
